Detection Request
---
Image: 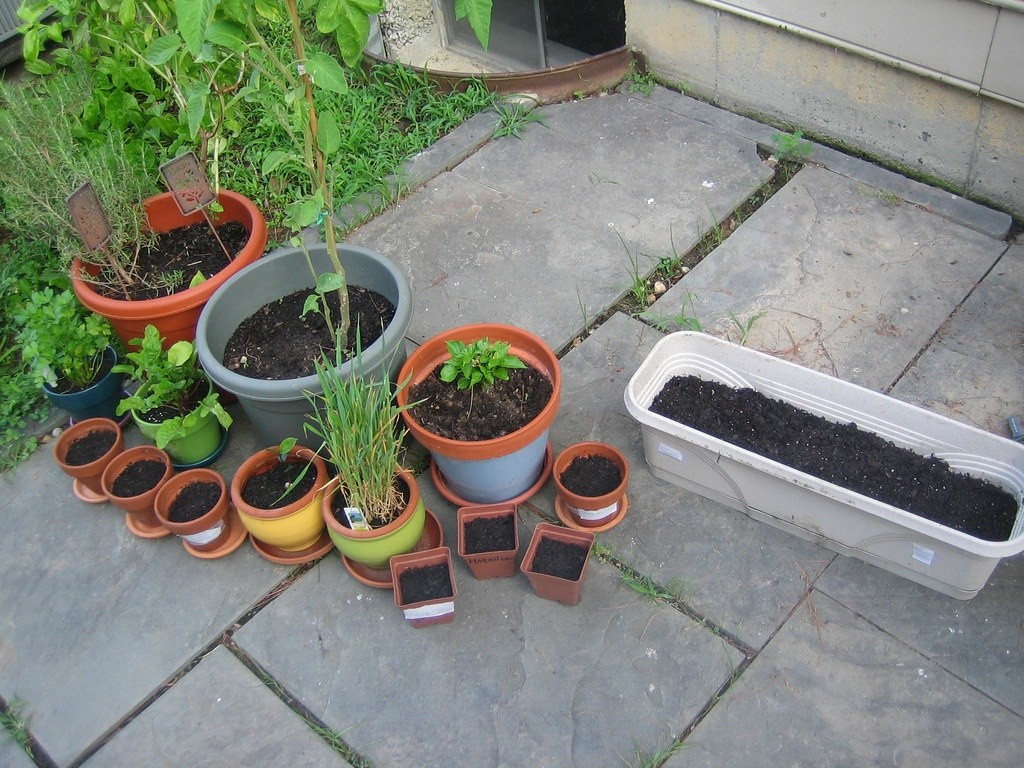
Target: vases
[230,441,335,565]
[389,546,457,629]
[623,330,1024,600]
[520,522,596,606]
[553,442,628,533]
[456,502,519,579]
[101,445,175,538]
[154,468,248,559]
[54,417,125,504]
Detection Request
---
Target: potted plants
[0,0,561,588]
